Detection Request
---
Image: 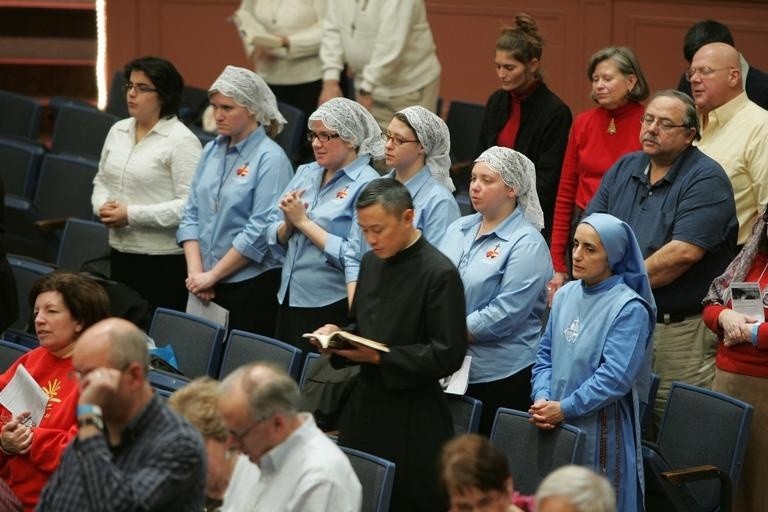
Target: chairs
[0,70,768,512]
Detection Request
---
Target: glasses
[381,131,418,145]
[125,80,158,92]
[686,67,733,80]
[306,131,340,142]
[639,115,687,130]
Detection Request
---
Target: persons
[0,1,768,512]
